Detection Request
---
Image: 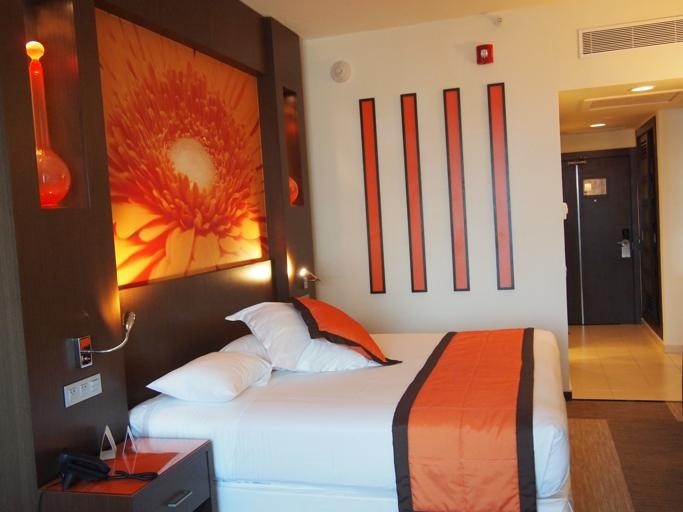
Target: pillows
[220,332,275,368]
[287,295,402,364]
[144,350,272,403]
[222,296,402,371]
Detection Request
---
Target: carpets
[568,398,682,510]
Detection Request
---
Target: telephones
[57,447,110,490]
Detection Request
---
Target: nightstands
[35,436,217,511]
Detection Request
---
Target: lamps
[299,267,320,291]
[70,309,138,369]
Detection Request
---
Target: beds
[127,325,572,511]
